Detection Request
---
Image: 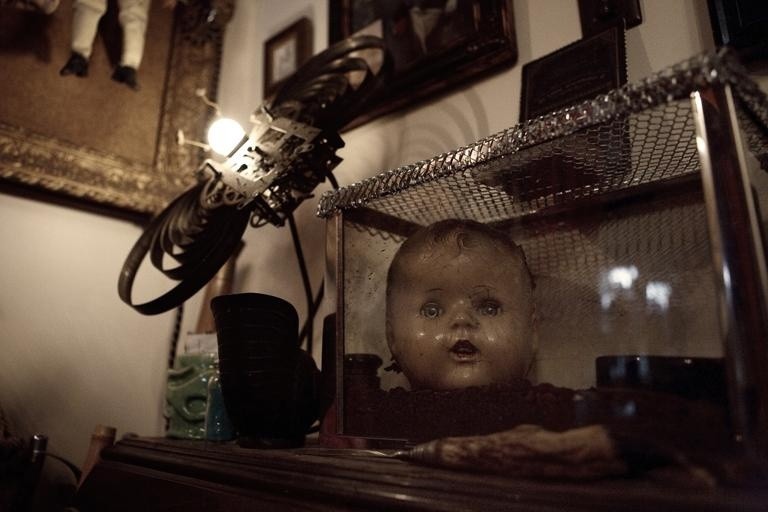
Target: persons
[60,0,150,88]
[385,219,540,394]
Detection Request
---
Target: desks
[96,429,766,509]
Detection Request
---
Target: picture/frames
[263,1,519,133]
[518,19,630,200]
[1,2,231,220]
[577,1,642,38]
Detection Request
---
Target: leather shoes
[110,66,138,87]
[60,52,88,77]
